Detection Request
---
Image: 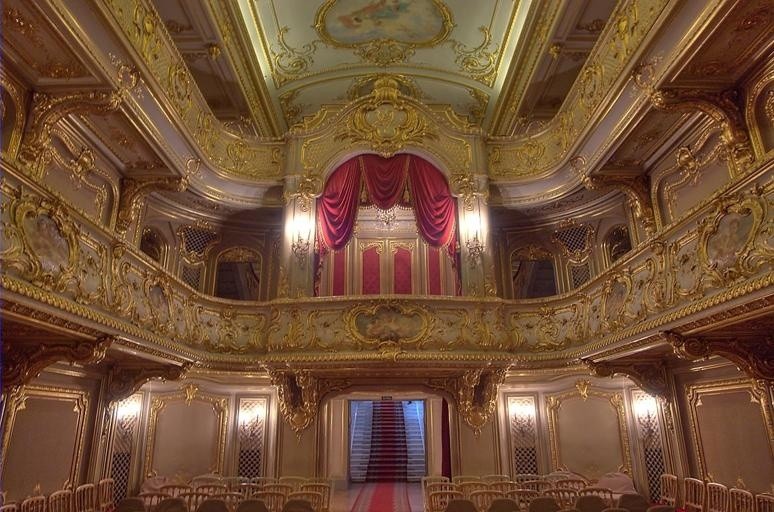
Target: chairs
[120,475,333,511]
[422,474,773,512]
[0,478,116,512]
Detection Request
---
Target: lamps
[291,231,310,269]
[465,237,486,269]
